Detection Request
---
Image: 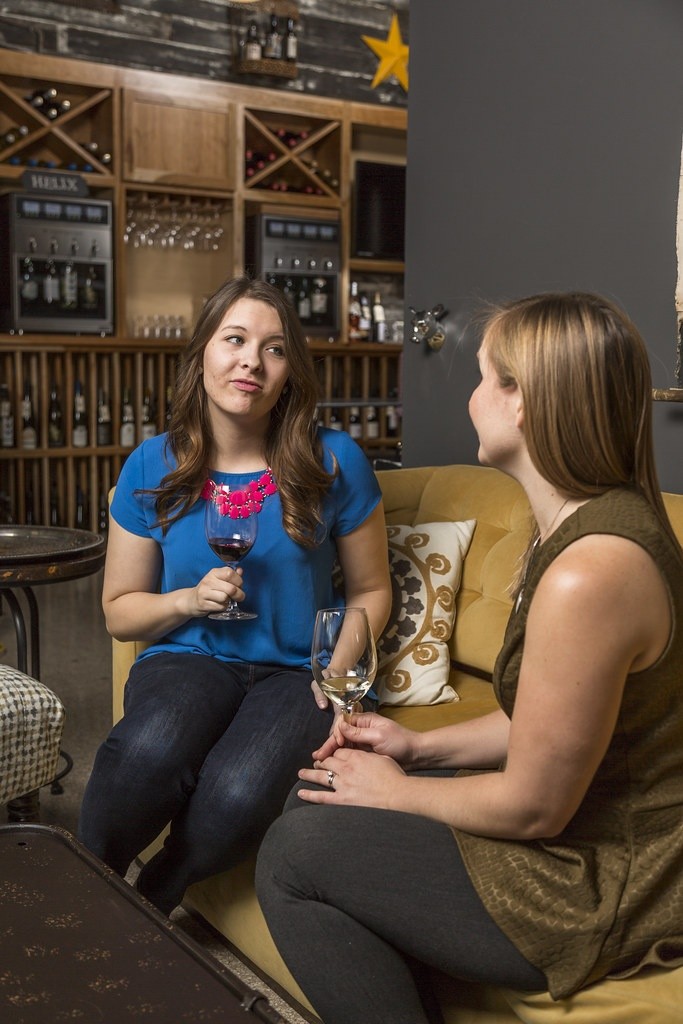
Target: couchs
[111,464,683,1024]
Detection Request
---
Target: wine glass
[123,196,223,251]
[204,486,258,620]
[312,607,377,748]
[132,312,184,339]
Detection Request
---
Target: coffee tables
[1,822,288,1024]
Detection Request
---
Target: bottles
[349,282,387,342]
[28,85,70,121]
[80,140,112,166]
[284,277,329,325]
[121,399,135,448]
[8,155,92,172]
[20,262,98,311]
[0,383,14,446]
[245,10,299,63]
[142,396,155,442]
[366,407,377,437]
[318,407,361,439]
[49,383,64,448]
[4,125,28,147]
[19,383,37,449]
[386,406,397,435]
[246,128,338,195]
[74,383,88,446]
[98,391,113,445]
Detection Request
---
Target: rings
[328,771,336,787]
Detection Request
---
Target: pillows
[331,518,479,708]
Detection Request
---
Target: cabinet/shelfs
[1,49,408,336]
[1,334,404,534]
[229,0,298,78]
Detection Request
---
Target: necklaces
[199,466,277,518]
[514,499,570,614]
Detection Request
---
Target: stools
[0,663,66,823]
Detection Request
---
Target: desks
[313,398,401,407]
[0,524,107,796]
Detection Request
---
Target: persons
[255,291,683,1024]
[81,276,392,918]
